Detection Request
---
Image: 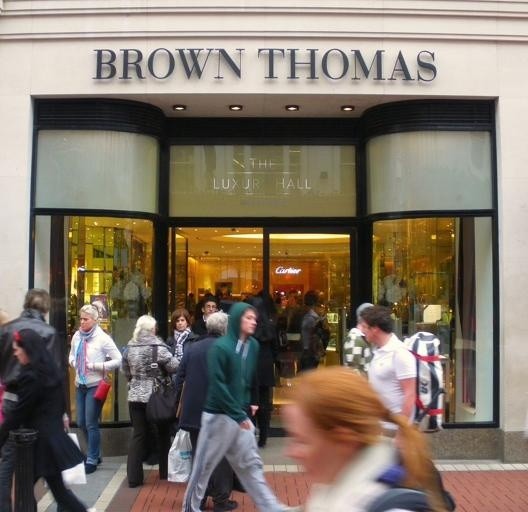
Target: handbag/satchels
[145,380,181,423]
[167,430,193,483]
[60,426,88,486]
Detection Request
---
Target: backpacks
[404,332,445,434]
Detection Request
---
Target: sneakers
[213,500,237,511]
[258,436,267,448]
[129,479,144,489]
[85,457,103,474]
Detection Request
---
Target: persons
[281,365,455,511]
[344,304,375,375]
[362,303,417,425]
[1,283,331,512]
